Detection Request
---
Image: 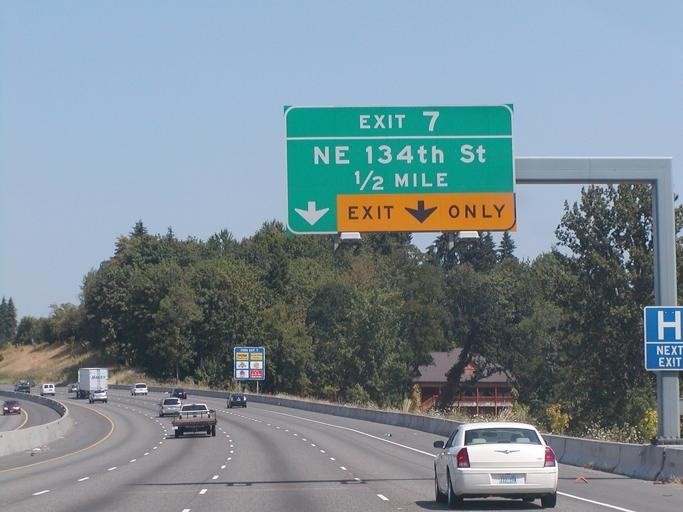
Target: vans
[39,383,56,397]
[14,379,31,395]
[157,397,181,416]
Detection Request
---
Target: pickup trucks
[171,403,219,437]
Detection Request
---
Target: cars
[226,391,248,409]
[130,382,148,395]
[432,420,559,508]
[169,388,187,399]
[89,389,108,403]
[67,384,77,392]
[1,400,21,415]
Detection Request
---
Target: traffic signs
[287,106,513,234]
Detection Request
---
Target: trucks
[75,367,108,398]
